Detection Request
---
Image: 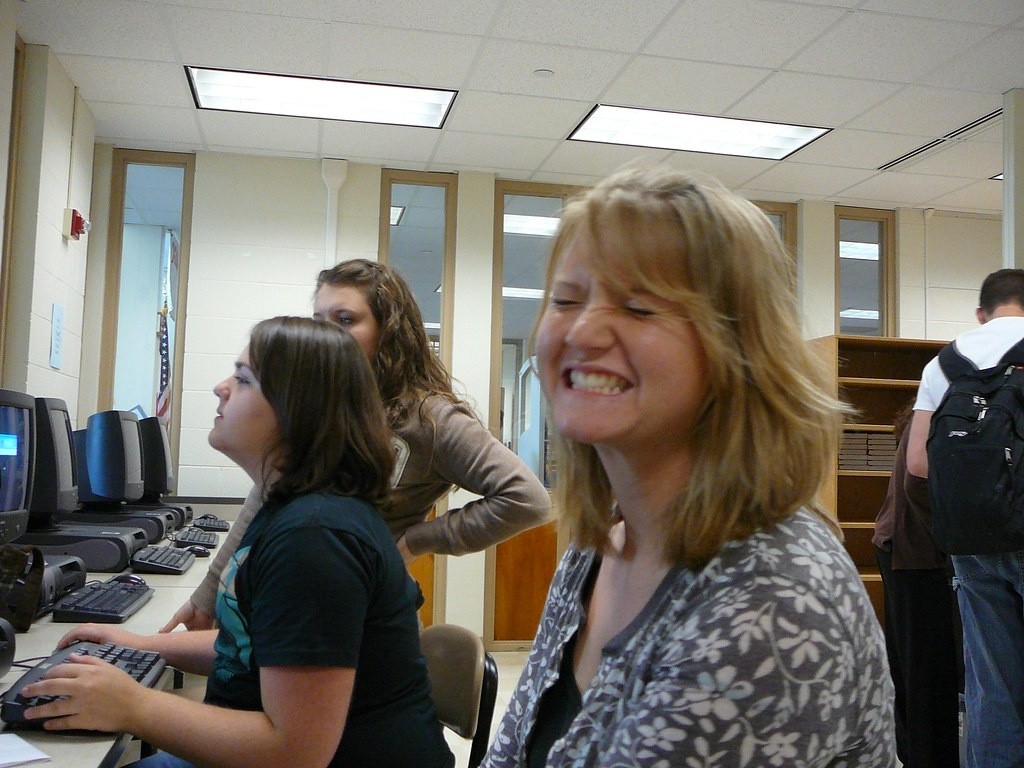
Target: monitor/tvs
[0,389,176,544]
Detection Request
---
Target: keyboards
[0,641,166,735]
[177,533,220,549]
[130,545,196,574]
[193,518,230,532]
[52,581,155,624]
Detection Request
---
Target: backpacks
[925,337,1024,555]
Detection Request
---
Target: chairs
[420,623,499,768]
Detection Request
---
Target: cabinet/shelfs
[802,334,951,613]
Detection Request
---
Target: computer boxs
[0,502,193,679]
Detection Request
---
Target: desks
[86,520,236,690]
[0,586,200,768]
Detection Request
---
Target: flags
[157,307,172,416]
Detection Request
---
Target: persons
[872,269,1024,768]
[477,167,903,768]
[160,259,554,704]
[20,316,454,768]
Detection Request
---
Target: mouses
[188,528,205,532]
[199,514,218,519]
[184,545,210,556]
[109,575,147,586]
